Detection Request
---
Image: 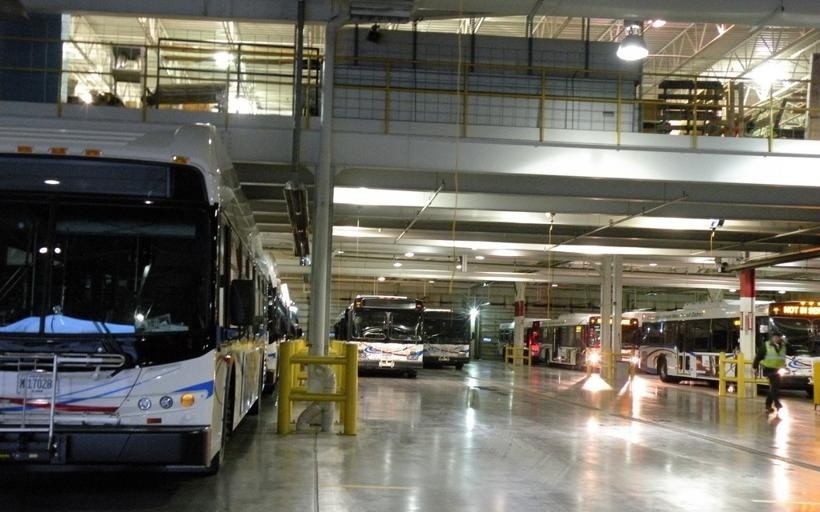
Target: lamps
[615,21,649,62]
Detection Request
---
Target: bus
[329,292,473,378]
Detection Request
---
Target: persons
[752,331,798,414]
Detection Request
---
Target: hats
[770,328,783,336]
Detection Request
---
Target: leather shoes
[775,400,782,411]
[765,404,774,412]
